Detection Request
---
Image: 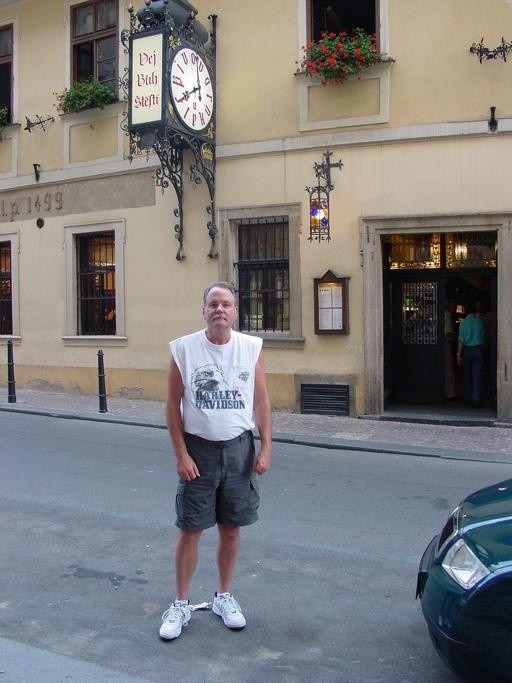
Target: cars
[415,479,512,683]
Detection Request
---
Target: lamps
[305,149,343,242]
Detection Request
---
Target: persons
[155,282,274,642]
[405,293,486,409]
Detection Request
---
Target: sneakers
[159,602,191,640]
[212,591,246,628]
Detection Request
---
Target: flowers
[299,25,384,82]
[55,76,116,116]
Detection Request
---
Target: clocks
[169,43,215,132]
